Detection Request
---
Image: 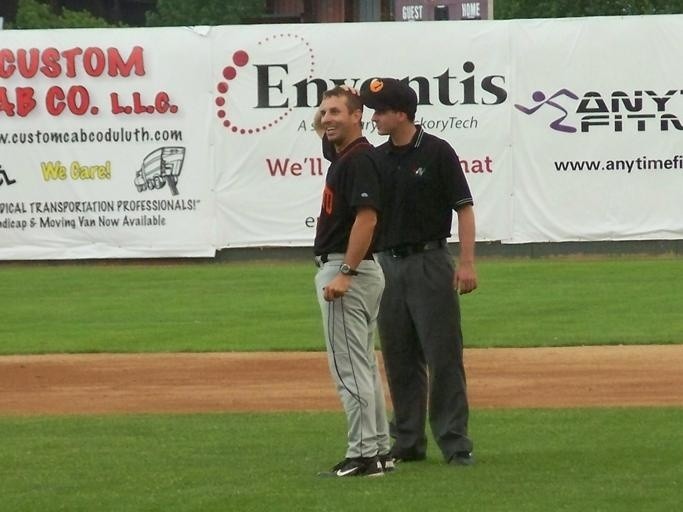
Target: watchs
[338,263,359,277]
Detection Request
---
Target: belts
[373,236,447,259]
[313,251,376,267]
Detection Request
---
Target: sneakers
[376,454,396,473]
[316,455,384,478]
[389,451,427,464]
[448,450,474,466]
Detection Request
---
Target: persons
[361,77,478,468]
[312,84,396,480]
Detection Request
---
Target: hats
[359,77,418,113]
